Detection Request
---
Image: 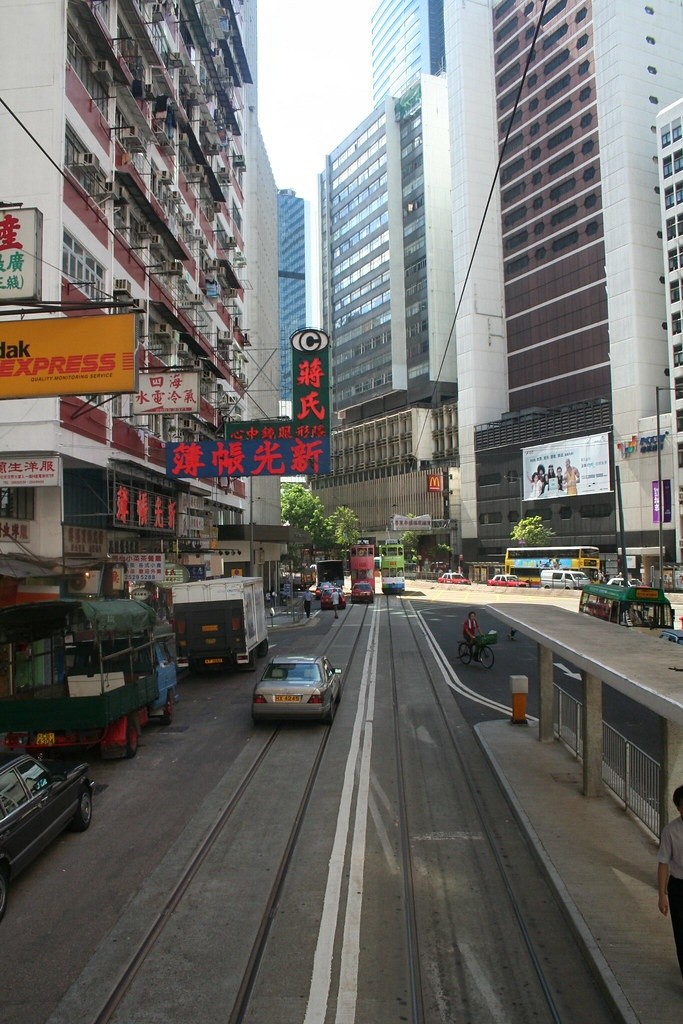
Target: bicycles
[457,634,495,669]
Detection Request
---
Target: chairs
[304,668,316,678]
[272,669,283,678]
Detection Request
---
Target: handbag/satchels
[338,595,342,604]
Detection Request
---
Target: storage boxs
[67,671,125,697]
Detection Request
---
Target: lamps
[392,504,398,507]
[178,539,242,556]
[342,504,348,507]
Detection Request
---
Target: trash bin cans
[280,591,288,606]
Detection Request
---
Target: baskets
[476,634,496,645]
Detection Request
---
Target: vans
[541,570,594,590]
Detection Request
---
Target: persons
[329,588,339,619]
[463,612,483,662]
[302,588,313,619]
[527,459,580,498]
[657,784,683,982]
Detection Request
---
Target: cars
[487,574,530,587]
[250,653,343,726]
[350,583,375,604]
[659,629,683,645]
[321,588,347,610]
[1,751,95,924]
[437,572,472,585]
[315,581,335,600]
[607,577,643,587]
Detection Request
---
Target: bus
[316,560,345,588]
[505,545,600,585]
[578,584,675,634]
[379,544,406,594]
[349,538,376,594]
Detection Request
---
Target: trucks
[171,575,269,673]
[0,597,179,762]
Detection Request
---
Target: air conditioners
[184,213,194,225]
[193,293,204,305]
[220,330,233,345]
[138,223,164,249]
[235,316,251,343]
[224,288,237,298]
[236,373,248,388]
[236,256,247,266]
[168,259,190,283]
[217,384,224,392]
[74,0,246,213]
[178,343,189,354]
[113,278,132,296]
[159,323,173,336]
[171,330,180,342]
[193,360,217,383]
[228,396,237,405]
[194,228,208,249]
[133,298,147,313]
[226,237,237,247]
[67,570,101,594]
[211,259,226,275]
